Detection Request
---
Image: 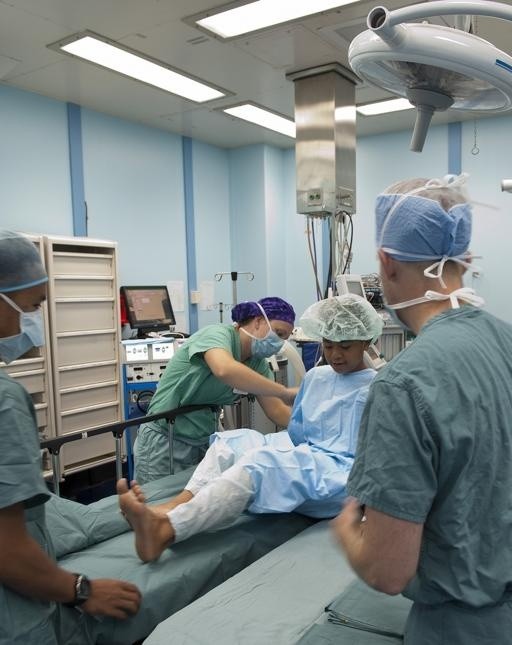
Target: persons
[117,295,381,562]
[133,297,303,487]
[329,178,512,645]
[0,228,142,645]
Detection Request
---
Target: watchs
[62,571,91,608]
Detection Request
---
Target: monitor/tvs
[120,285,177,339]
[365,287,383,308]
[335,274,367,301]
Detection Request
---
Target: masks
[0,294,45,365]
[251,318,285,359]
[382,303,412,332]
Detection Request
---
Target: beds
[49,452,419,644]
